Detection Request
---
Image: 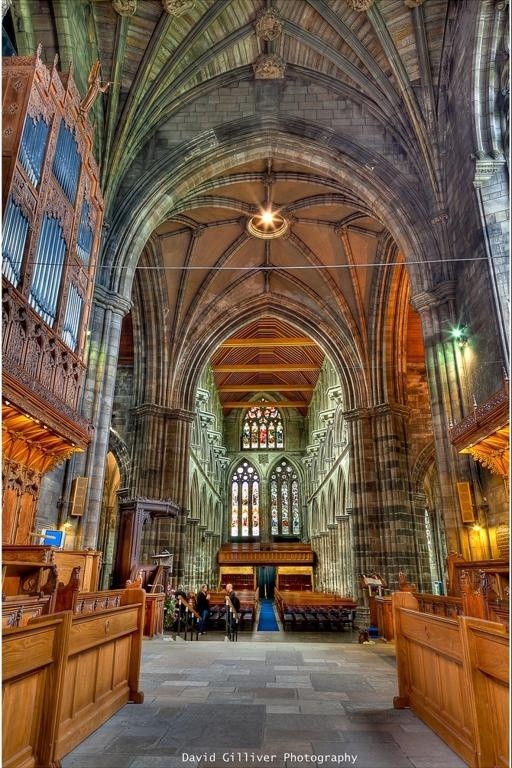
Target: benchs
[273,587,358,631]
[208,591,258,631]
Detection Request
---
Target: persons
[195,584,211,635]
[224,583,240,640]
